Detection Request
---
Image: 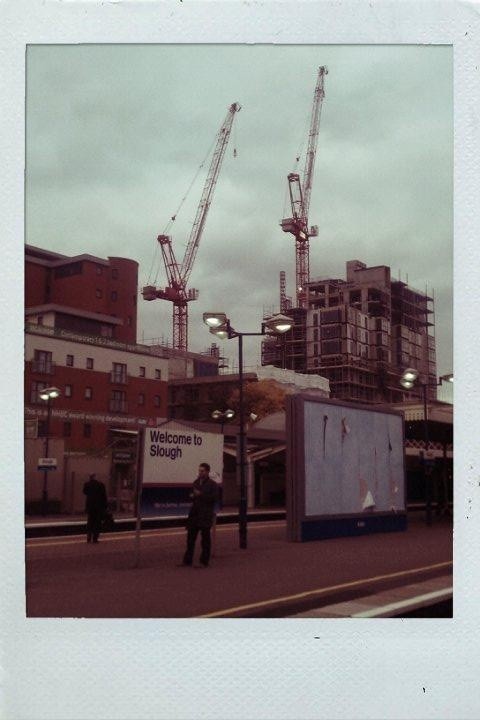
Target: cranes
[279,64,329,308]
[139,101,243,351]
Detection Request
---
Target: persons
[82,470,109,545]
[181,462,222,570]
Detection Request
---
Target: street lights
[201,311,295,548]
[212,409,235,434]
[400,367,453,450]
[39,386,61,514]
